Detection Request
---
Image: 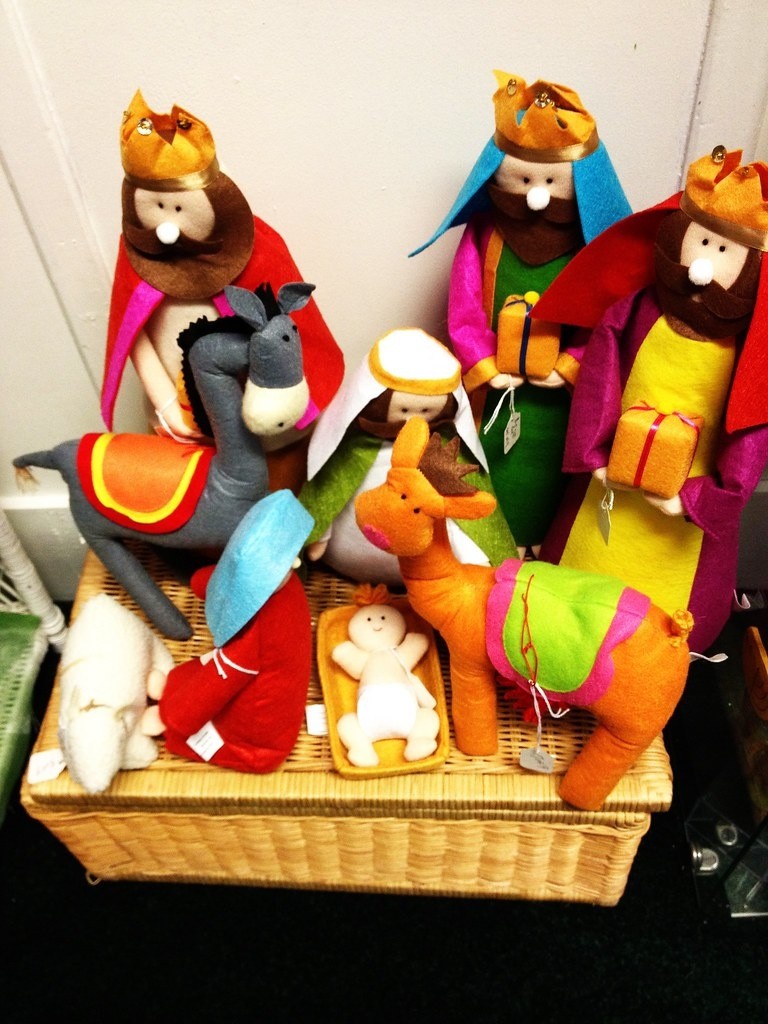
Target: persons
[497,146,768,724]
[408,69,634,561]
[101,90,345,499]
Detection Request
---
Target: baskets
[17,538,673,907]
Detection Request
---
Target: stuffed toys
[332,605,439,766]
[298,328,522,594]
[11,277,316,640]
[159,489,316,772]
[355,417,694,811]
[57,594,176,794]
